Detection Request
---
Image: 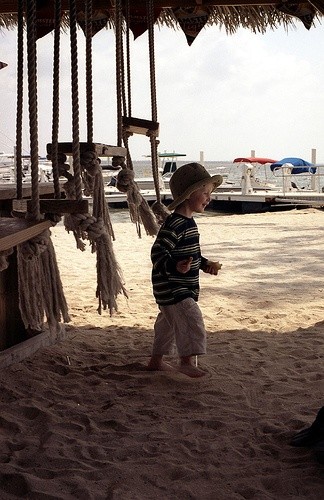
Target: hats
[168,163,223,210]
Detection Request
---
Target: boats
[0,144,324,203]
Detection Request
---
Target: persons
[145,162,224,379]
